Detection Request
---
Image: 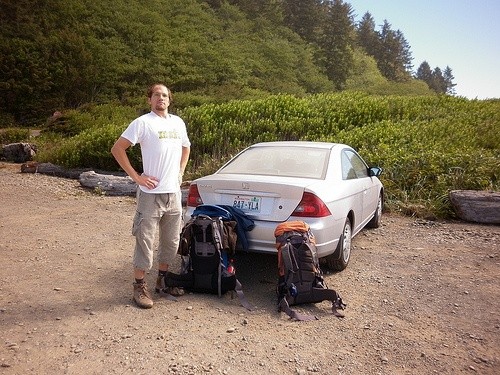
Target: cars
[184,141,386,271]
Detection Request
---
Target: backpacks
[274,219,347,320]
[179,219,237,295]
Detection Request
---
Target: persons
[110,85,191,308]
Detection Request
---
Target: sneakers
[155,275,187,296]
[131,282,153,308]
